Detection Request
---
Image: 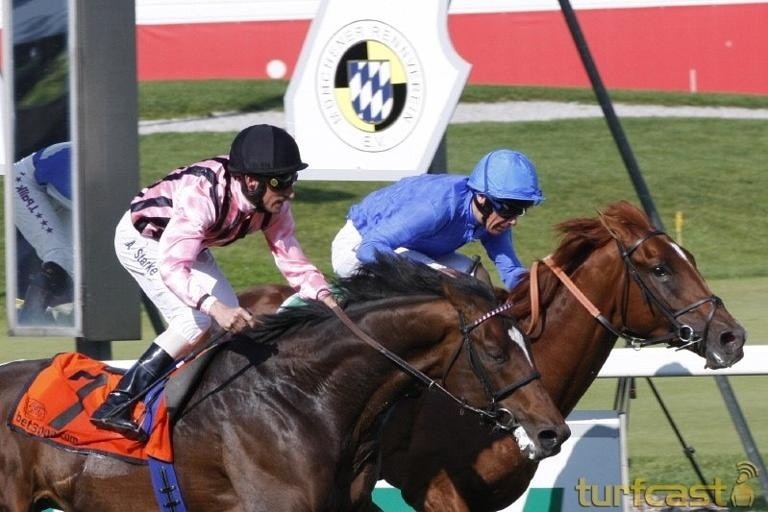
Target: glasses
[249,172,300,192]
[487,200,527,220]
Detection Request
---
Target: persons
[90,124,330,442]
[330,150,547,292]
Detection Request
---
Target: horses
[0,239,573,512]
[189,196,749,512]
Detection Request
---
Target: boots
[87,340,178,441]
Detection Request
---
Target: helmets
[466,148,544,204]
[226,123,309,178]
